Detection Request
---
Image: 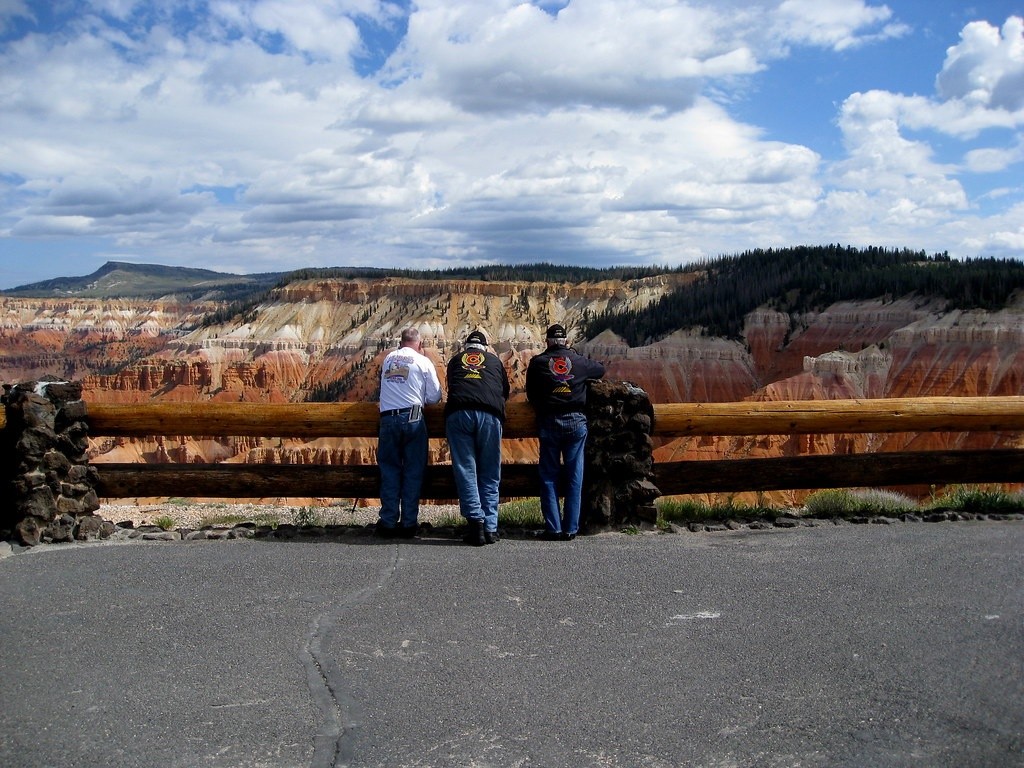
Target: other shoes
[538,532,562,541]
[376,522,398,539]
[399,526,415,539]
[563,533,576,540]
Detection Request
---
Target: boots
[485,532,500,544]
[463,518,486,546]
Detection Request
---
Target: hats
[465,331,488,346]
[547,324,566,338]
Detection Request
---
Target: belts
[380,408,412,416]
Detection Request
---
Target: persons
[526,324,606,541]
[376,327,443,536]
[443,330,511,546]
